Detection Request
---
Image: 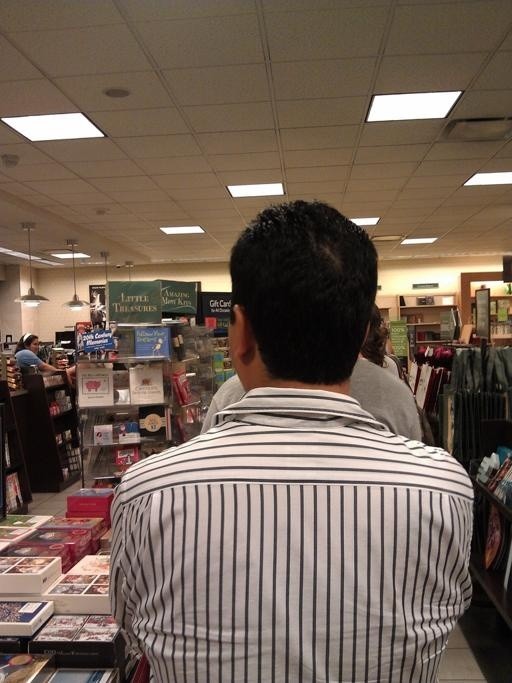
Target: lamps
[14,221,110,312]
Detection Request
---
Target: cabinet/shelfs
[0,320,236,514]
[396,270,512,630]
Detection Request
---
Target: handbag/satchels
[409,344,454,418]
[439,347,512,459]
[385,352,436,447]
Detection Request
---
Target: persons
[196,331,427,448]
[349,302,401,376]
[14,332,60,379]
[103,197,480,683]
